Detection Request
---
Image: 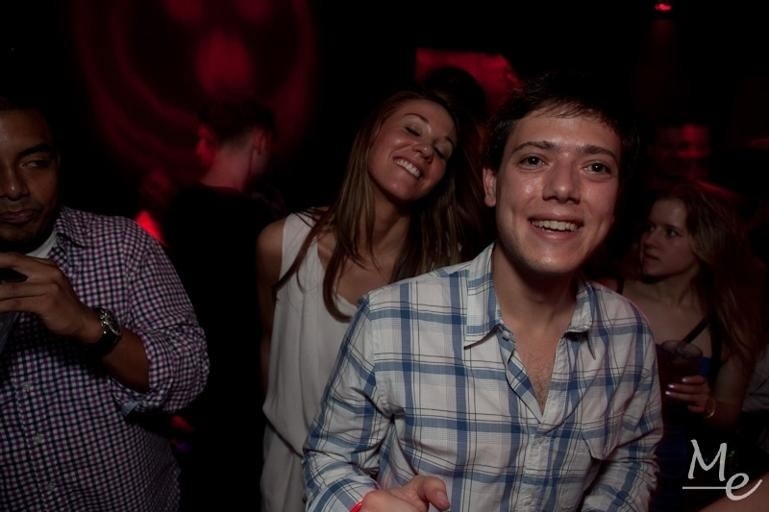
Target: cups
[656,338,703,410]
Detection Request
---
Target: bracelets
[76,304,122,365]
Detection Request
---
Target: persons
[299,68,666,512]
[131,102,244,212]
[417,62,504,268]
[127,96,287,512]
[593,104,768,512]
[1,85,210,511]
[246,86,462,511]
